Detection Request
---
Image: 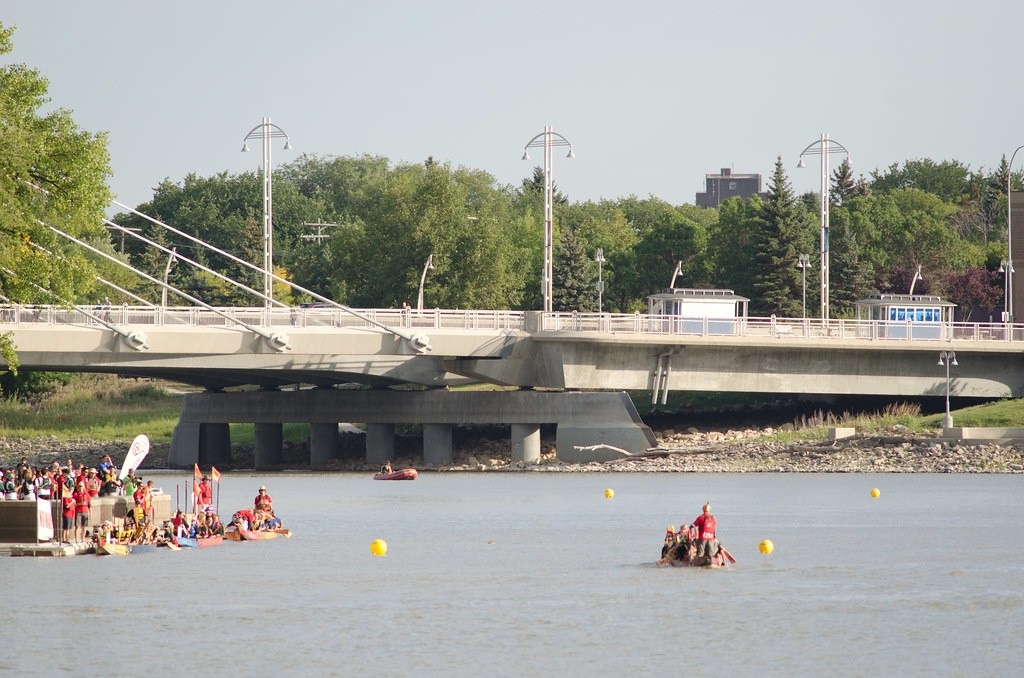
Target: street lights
[670,260,683,288]
[908,265,922,295]
[936,350,960,429]
[521,125,575,319]
[796,133,855,328]
[164,246,177,306]
[240,116,293,325]
[418,253,436,318]
[796,253,812,336]
[997,258,1015,344]
[594,248,605,334]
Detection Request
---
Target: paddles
[716,538,735,564]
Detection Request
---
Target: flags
[194,462,203,480]
[194,477,201,496]
[212,466,220,481]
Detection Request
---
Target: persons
[1,297,113,324]
[1,454,280,549]
[664,504,716,559]
[381,460,394,475]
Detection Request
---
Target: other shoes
[62,541,70,544]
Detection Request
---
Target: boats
[161,522,226,548]
[91,523,158,557]
[233,513,285,539]
[373,468,418,481]
[661,524,727,571]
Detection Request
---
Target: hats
[259,485,265,489]
[6,474,14,480]
[88,468,98,473]
[702,504,710,512]
[201,476,211,481]
[68,472,75,477]
[53,463,60,467]
[79,481,84,487]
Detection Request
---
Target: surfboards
[118,434,149,480]
